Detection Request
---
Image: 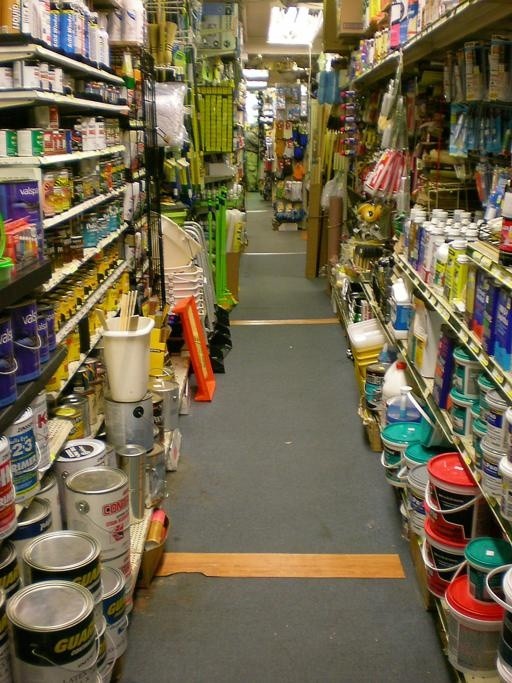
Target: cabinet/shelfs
[327,0,512,683]
[0,41,191,554]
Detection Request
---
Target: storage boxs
[334,0,365,40]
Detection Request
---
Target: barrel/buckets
[397,443,458,481]
[9,496,52,584]
[96,616,117,682]
[363,361,388,415]
[53,438,107,522]
[0,438,19,537]
[470,404,481,420]
[0,538,23,598]
[484,563,512,683]
[149,380,180,431]
[104,393,154,453]
[472,421,486,467]
[100,563,129,663]
[145,444,169,506]
[424,453,483,548]
[444,563,503,676]
[32,389,53,467]
[6,579,96,683]
[102,550,134,614]
[22,529,103,633]
[147,366,176,385]
[480,438,502,489]
[463,538,510,605]
[0,585,11,683]
[7,409,40,500]
[36,469,62,530]
[497,459,512,520]
[421,532,468,602]
[64,466,131,561]
[448,388,471,436]
[405,391,456,448]
[484,389,507,445]
[453,345,481,394]
[408,465,427,535]
[380,422,422,488]
[477,372,494,411]
